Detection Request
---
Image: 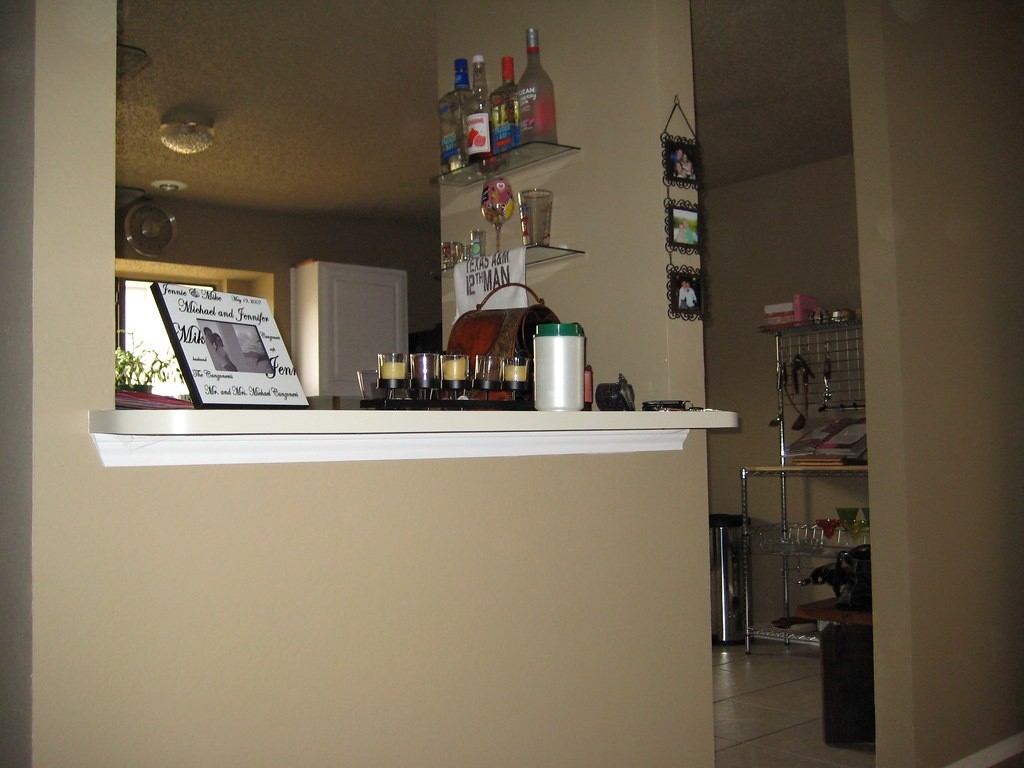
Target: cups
[378,352,529,381]
[441,230,485,269]
[518,188,552,247]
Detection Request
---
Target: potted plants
[114,329,184,394]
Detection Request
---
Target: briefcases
[445,282,562,402]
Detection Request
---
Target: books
[785,416,868,455]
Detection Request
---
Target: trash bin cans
[710,512,751,647]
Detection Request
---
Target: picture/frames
[670,270,705,316]
[150,281,311,409]
[668,205,701,250]
[665,139,702,185]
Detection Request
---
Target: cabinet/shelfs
[429,141,587,278]
[739,315,868,654]
[290,262,408,396]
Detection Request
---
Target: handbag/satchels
[832,544,872,610]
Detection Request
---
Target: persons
[679,280,697,310]
[204,326,238,371]
[671,146,695,180]
[675,220,698,244]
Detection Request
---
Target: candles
[440,355,469,380]
[409,353,440,379]
[474,355,504,381]
[377,352,408,379]
[503,358,530,381]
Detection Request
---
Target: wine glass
[816,518,842,556]
[839,519,869,550]
[481,178,514,251]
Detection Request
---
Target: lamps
[160,112,215,154]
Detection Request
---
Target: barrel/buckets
[533,324,585,412]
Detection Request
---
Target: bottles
[438,28,558,186]
